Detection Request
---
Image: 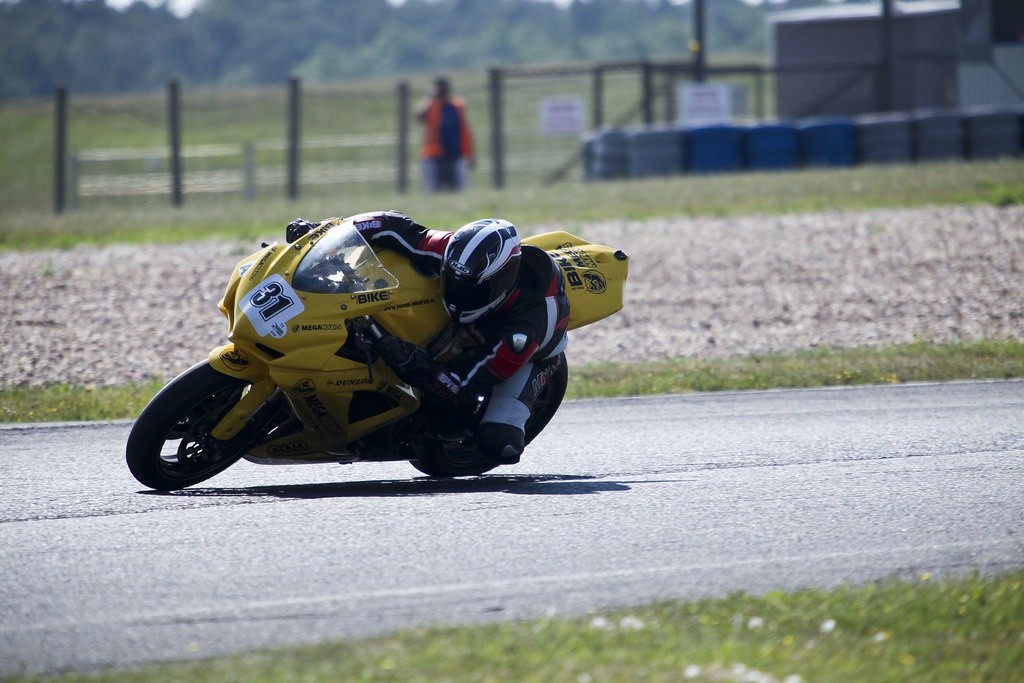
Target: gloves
[287,218,321,243]
[376,336,410,365]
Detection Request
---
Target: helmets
[441,218,521,324]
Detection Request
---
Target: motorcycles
[125,215,631,493]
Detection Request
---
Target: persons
[412,78,480,196]
[287,215,571,464]
